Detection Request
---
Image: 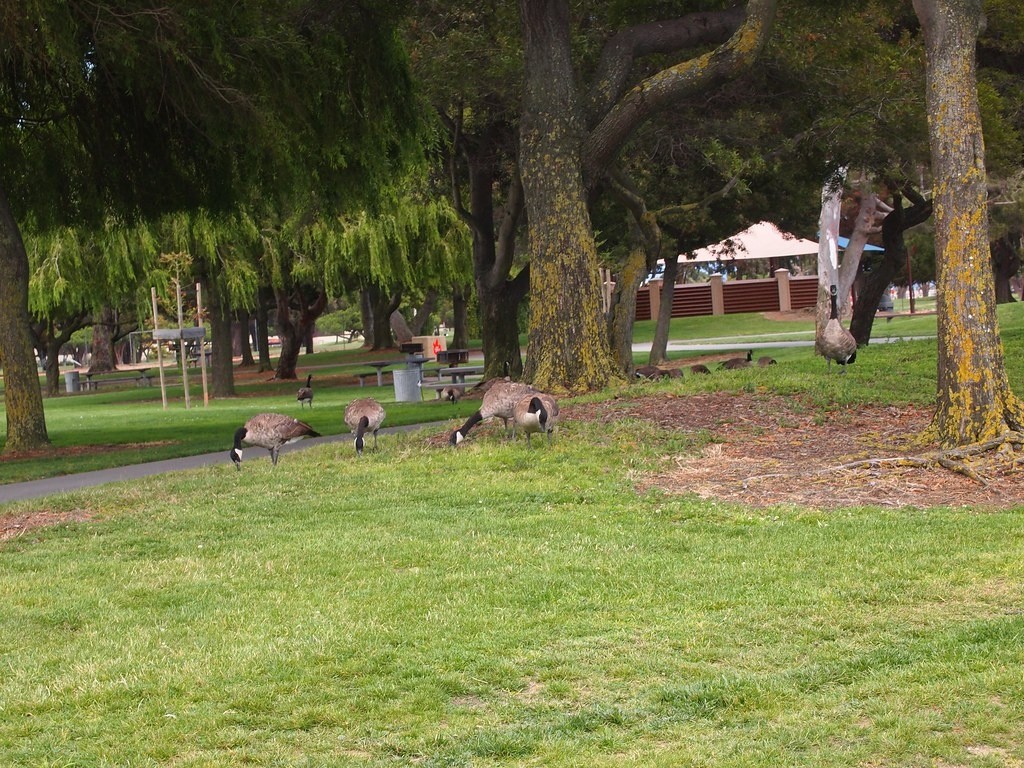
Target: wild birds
[450,378,561,448]
[297,374,313,410]
[443,386,462,406]
[717,349,777,370]
[817,285,857,378]
[690,364,711,376]
[634,365,684,381]
[344,397,387,458]
[229,414,323,473]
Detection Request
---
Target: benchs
[427,383,479,399]
[78,376,155,392]
[422,368,446,379]
[354,370,393,387]
[189,359,197,368]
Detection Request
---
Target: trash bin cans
[393,368,422,401]
[64,371,80,392]
[194,353,213,367]
[877,289,893,312]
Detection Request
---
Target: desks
[404,358,435,381]
[81,368,151,391]
[439,366,485,393]
[195,352,211,366]
[364,360,392,387]
